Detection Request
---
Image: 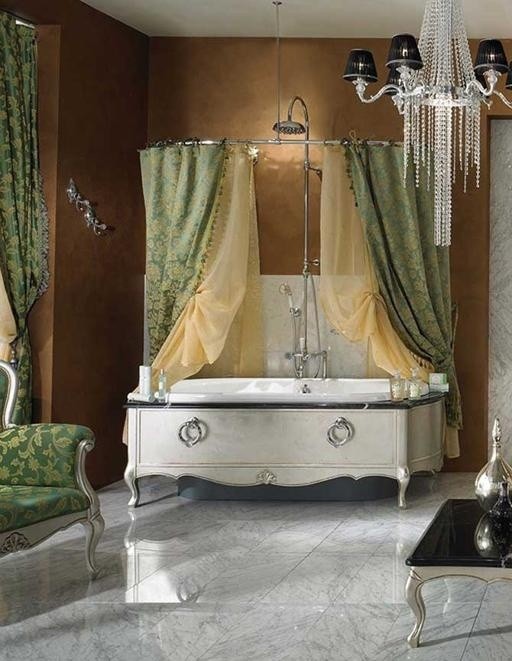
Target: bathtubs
[165,376,391,403]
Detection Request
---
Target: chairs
[2,362,106,582]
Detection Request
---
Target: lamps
[344,0,511,247]
